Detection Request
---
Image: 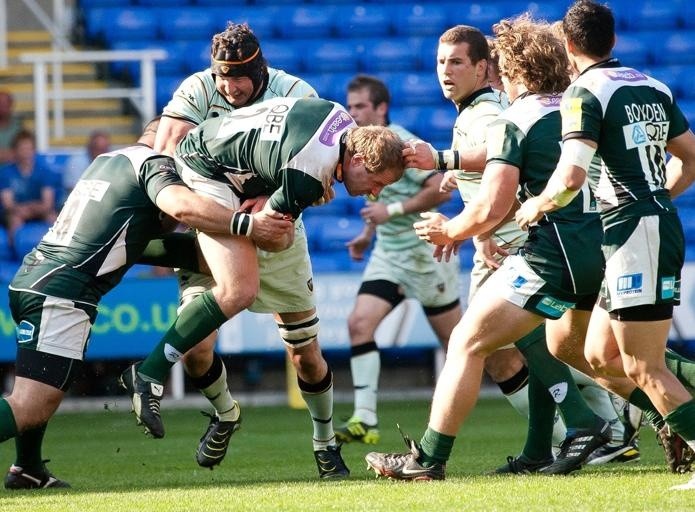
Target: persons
[116,97,408,439]
[0,115,293,490]
[362,1,694,488]
[0,92,24,165]
[154,23,354,482]
[0,130,59,260]
[323,77,569,446]
[59,129,113,197]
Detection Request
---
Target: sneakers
[116,361,166,439]
[4,460,71,489]
[197,400,242,466]
[315,416,445,481]
[495,417,695,474]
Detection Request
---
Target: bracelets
[229,211,253,238]
